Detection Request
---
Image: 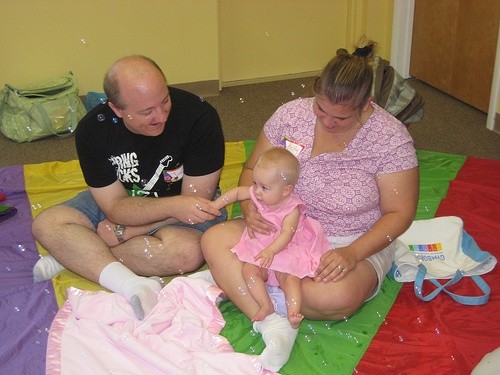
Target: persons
[199,39,420,372]
[31,54,225,321]
[208,146,333,328]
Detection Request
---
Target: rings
[338,266,343,271]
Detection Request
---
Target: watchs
[113,224,126,243]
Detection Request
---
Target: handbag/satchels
[1,70,88,143]
[386,215,497,306]
